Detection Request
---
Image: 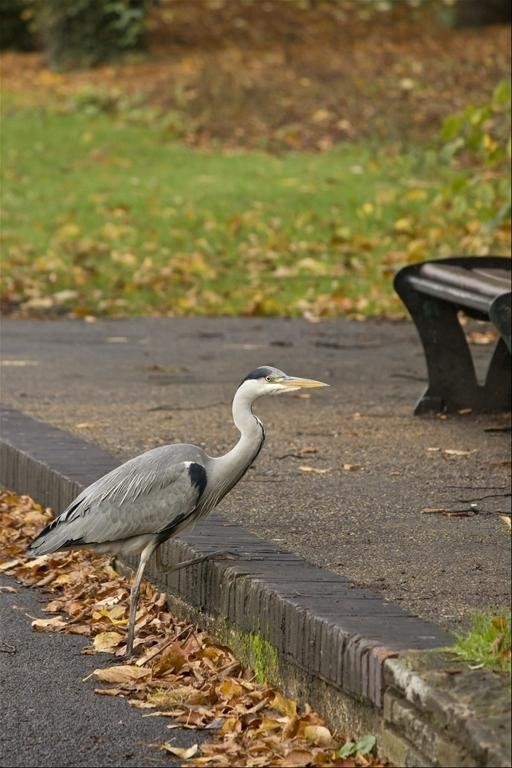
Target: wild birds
[26,364,333,663]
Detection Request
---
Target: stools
[393,255,512,418]
